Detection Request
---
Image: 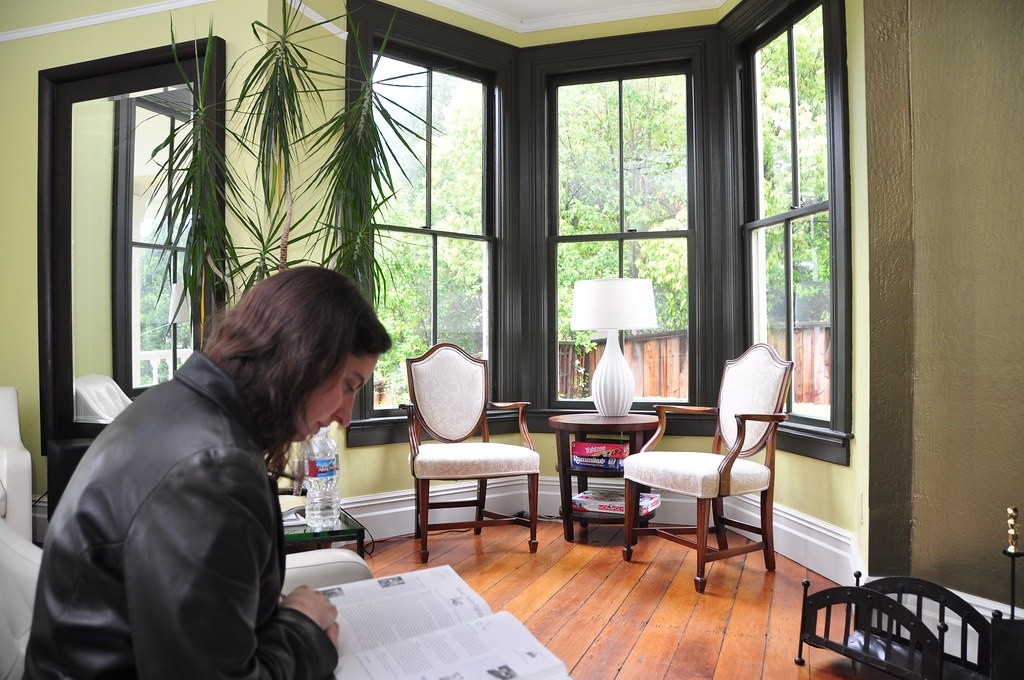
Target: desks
[548,413,659,546]
[277,507,366,560]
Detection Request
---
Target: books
[301,563,571,679]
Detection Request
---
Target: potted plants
[125,0,449,555]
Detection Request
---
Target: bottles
[303,428,341,528]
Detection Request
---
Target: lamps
[570,277,658,418]
[168,283,189,324]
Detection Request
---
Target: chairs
[73,373,133,424]
[621,343,794,595]
[0,516,374,680]
[398,343,540,563]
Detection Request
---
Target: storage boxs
[572,490,661,517]
[571,438,630,470]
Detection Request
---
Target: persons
[24,264,391,680]
